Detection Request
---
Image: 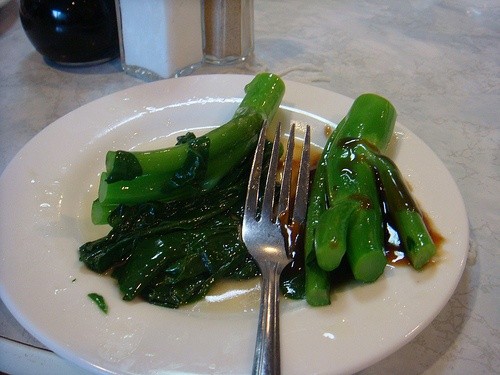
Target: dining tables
[0,0,499,374]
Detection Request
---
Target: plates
[0,71,472,375]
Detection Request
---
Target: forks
[241,120,312,375]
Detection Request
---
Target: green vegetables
[78,72,436,314]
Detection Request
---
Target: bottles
[113,0,208,85]
[199,0,253,66]
[18,0,119,69]
